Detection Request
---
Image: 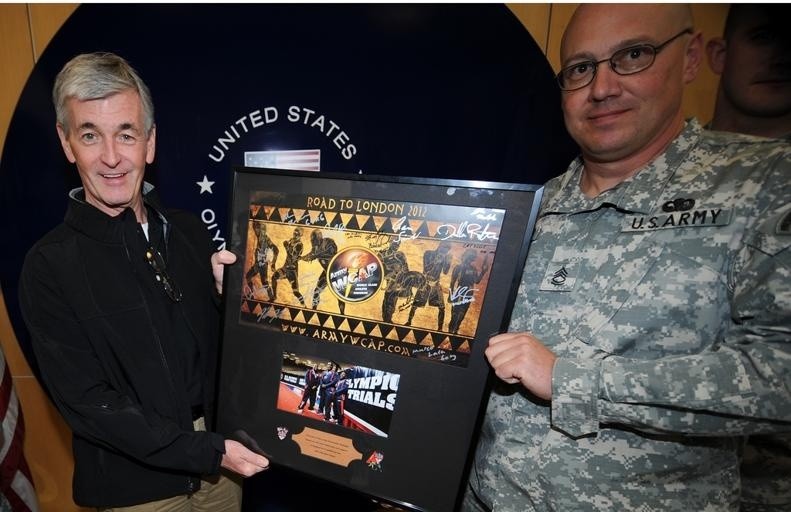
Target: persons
[269,226,306,306]
[331,370,349,426]
[404,240,452,334]
[299,228,345,315]
[17,50,272,511]
[245,221,281,302]
[316,361,339,420]
[376,236,426,324]
[451,3,791,511]
[703,3,791,512]
[299,362,320,410]
[447,246,490,334]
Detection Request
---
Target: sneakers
[298,407,342,427]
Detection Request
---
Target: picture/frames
[217,163,546,512]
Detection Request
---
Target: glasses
[556,30,692,92]
[144,248,183,303]
